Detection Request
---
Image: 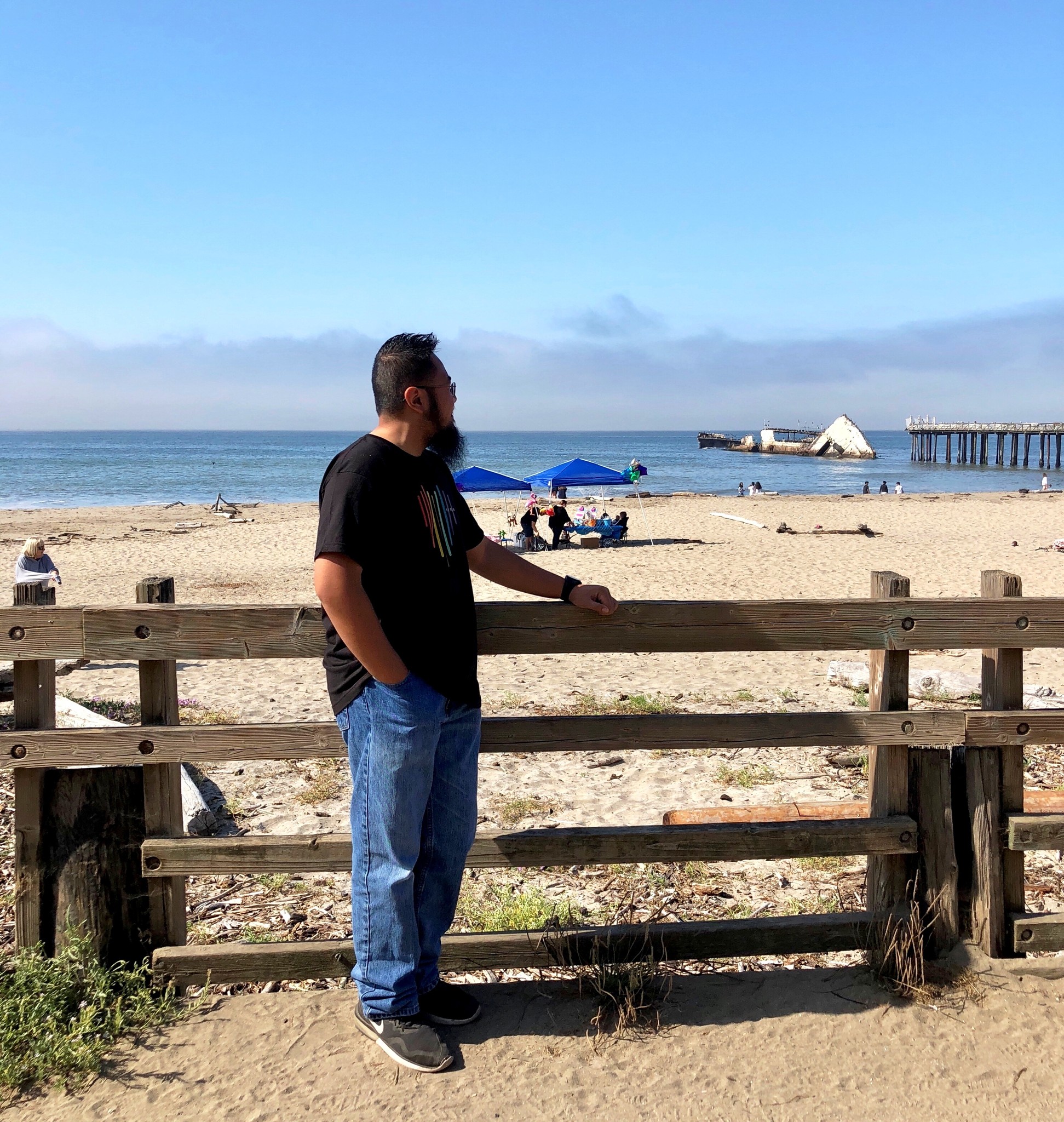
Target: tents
[452,466,549,552]
[509,457,654,547]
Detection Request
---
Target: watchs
[560,574,582,602]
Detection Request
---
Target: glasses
[403,383,456,401]
[37,545,44,550]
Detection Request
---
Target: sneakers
[418,980,482,1024]
[352,997,454,1073]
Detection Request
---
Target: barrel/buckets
[596,519,603,526]
[587,519,595,527]
[603,519,612,526]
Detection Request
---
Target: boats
[696,429,742,450]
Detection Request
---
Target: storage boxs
[581,537,599,548]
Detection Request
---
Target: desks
[564,525,623,549]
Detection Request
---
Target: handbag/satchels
[599,534,615,547]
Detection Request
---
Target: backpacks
[517,530,531,549]
[1019,489,1030,493]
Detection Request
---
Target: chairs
[618,527,628,545]
[498,531,513,549]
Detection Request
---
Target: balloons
[620,457,641,482]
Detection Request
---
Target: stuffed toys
[525,493,537,516]
[506,509,518,527]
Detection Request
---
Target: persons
[548,500,575,550]
[520,506,547,552]
[613,511,627,526]
[16,538,62,587]
[548,480,567,499]
[313,333,617,1075]
